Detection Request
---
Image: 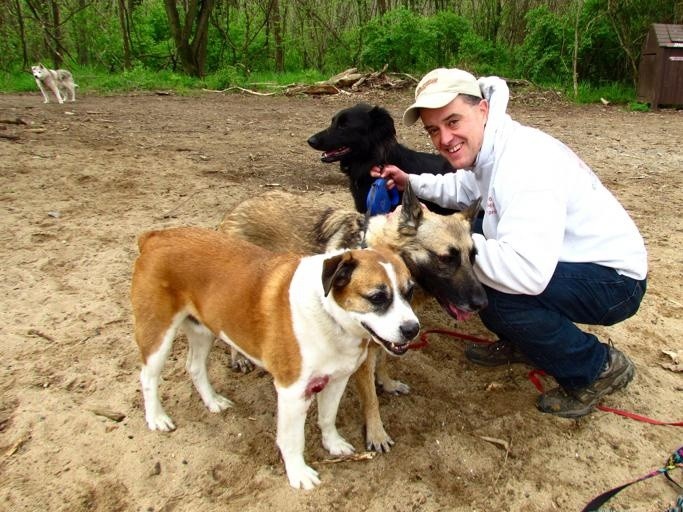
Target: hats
[401,64,484,127]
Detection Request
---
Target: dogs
[219,175,489,455]
[306,101,486,219]
[31,63,79,104]
[131,224,422,492]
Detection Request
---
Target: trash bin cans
[636,23,683,111]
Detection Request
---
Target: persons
[370,64,648,416]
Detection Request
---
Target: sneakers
[535,343,637,417]
[465,340,535,368]
